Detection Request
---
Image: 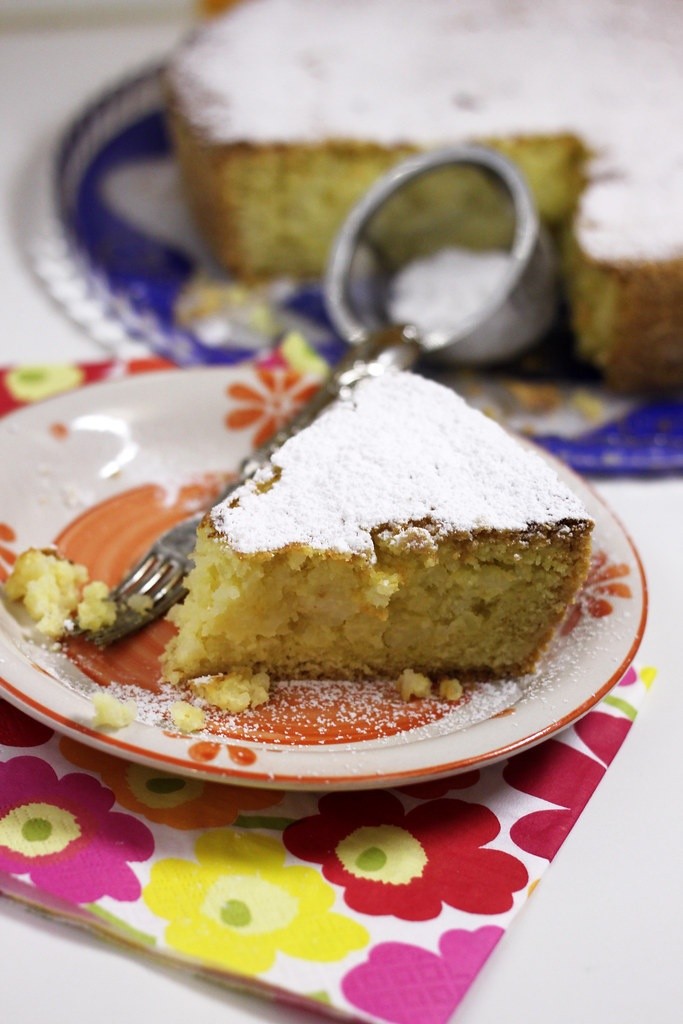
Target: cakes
[156,369,595,713]
[167,0,683,397]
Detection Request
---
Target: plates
[26,50,682,483]
[0,365,649,791]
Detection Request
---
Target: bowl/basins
[324,148,559,367]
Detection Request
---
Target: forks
[65,321,439,647]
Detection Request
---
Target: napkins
[0,357,655,1024]
